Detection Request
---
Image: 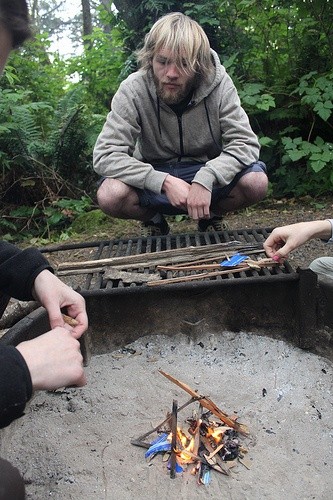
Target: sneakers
[140,216,170,237]
[198,216,230,232]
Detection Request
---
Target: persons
[93,12,268,236]
[263,219,333,300]
[0,0,88,500]
